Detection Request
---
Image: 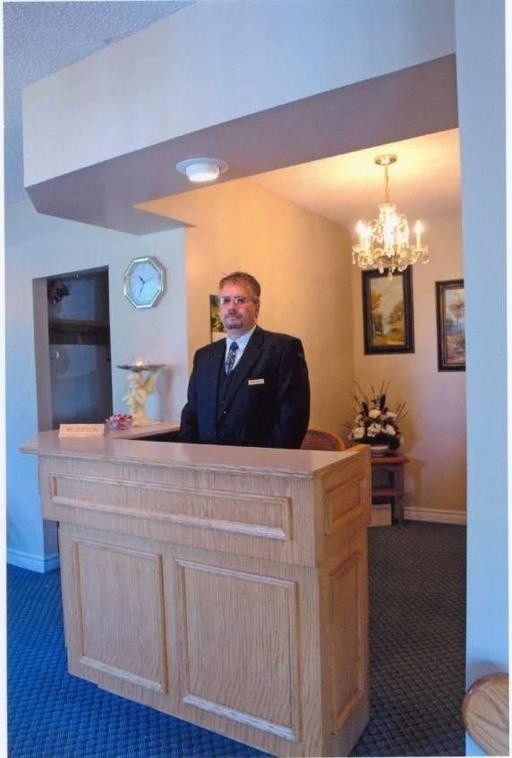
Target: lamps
[351,155,429,277]
[176,158,227,183]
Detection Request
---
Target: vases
[371,445,387,456]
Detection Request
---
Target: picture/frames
[434,279,465,371]
[361,264,415,353]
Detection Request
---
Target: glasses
[220,295,248,305]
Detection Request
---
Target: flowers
[344,377,408,449]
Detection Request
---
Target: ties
[225,341,238,376]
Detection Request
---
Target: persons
[177,271,311,449]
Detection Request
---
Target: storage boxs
[370,505,392,527]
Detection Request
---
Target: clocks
[124,256,166,308]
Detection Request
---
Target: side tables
[369,452,410,531]
